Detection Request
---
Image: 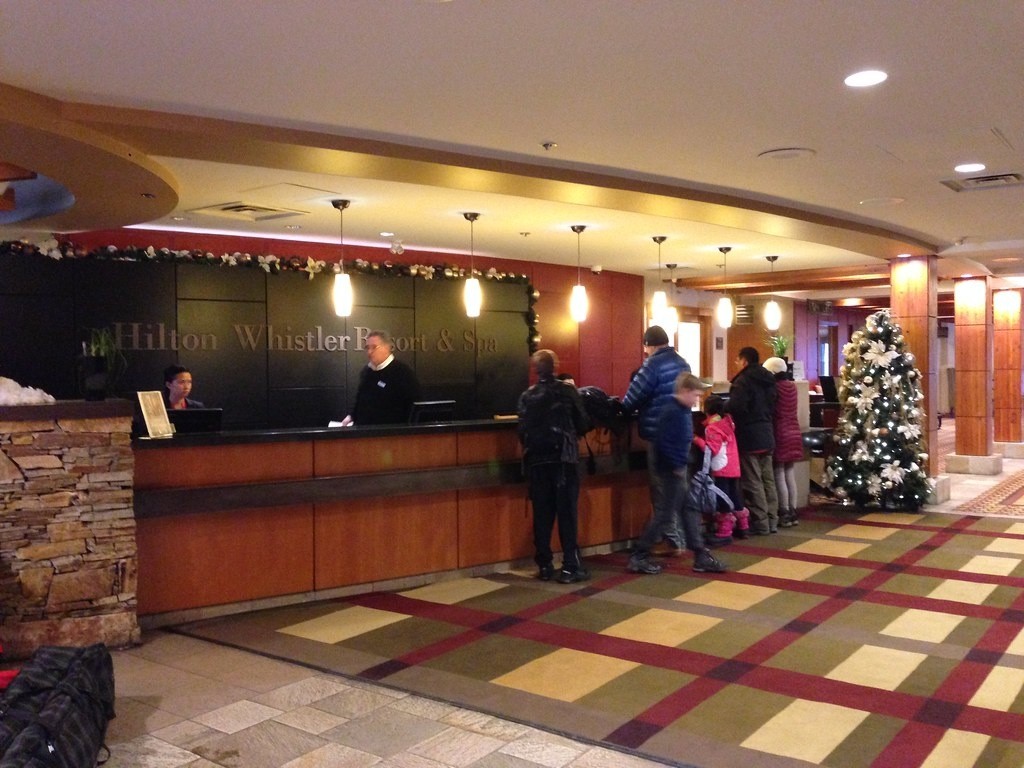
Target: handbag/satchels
[0,643,117,768]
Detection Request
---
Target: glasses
[364,344,383,351]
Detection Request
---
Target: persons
[518,350,592,583]
[693,396,749,544]
[724,347,804,535]
[621,326,691,552]
[558,374,575,385]
[626,374,728,572]
[342,332,417,426]
[163,365,204,409]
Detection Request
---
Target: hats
[643,325,669,346]
[762,357,787,375]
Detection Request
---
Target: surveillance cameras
[591,265,602,275]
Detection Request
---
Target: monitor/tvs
[409,400,456,424]
[167,408,223,433]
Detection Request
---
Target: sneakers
[626,552,662,574]
[539,564,554,580]
[692,548,729,572]
[559,567,591,583]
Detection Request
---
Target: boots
[706,512,737,547]
[733,507,750,540]
[778,509,792,527]
[790,507,799,525]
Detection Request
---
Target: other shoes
[769,524,778,532]
[735,528,770,536]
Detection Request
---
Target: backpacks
[688,445,734,514]
[576,386,631,438]
